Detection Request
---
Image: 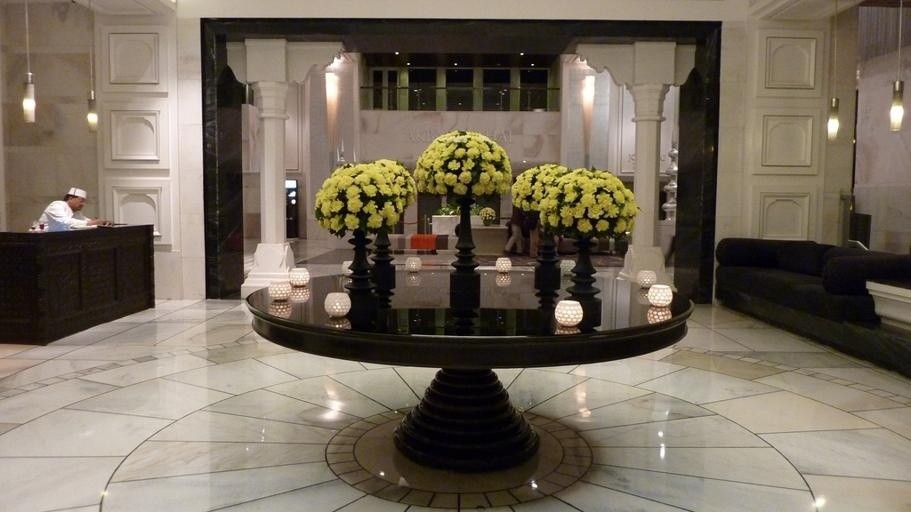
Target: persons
[499,203,527,255]
[24,186,115,233]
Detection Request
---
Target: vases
[448,197,481,268]
[535,225,560,263]
[565,271,601,327]
[370,265,395,307]
[370,218,396,265]
[535,268,561,311]
[565,238,600,295]
[450,267,481,317]
[483,220,492,225]
[345,228,378,290]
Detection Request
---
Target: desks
[470,225,510,256]
[247,271,694,474]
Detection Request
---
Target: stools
[405,233,448,254]
[365,233,407,254]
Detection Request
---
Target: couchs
[715,238,911,379]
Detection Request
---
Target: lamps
[826,0,840,141]
[22,0,37,124]
[890,0,905,132]
[86,0,98,135]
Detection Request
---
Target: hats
[67,187,87,201]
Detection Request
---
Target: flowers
[477,207,497,220]
[511,164,571,213]
[373,159,418,209]
[413,130,512,197]
[540,168,637,243]
[314,162,402,237]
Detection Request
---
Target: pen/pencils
[95,217,97,219]
